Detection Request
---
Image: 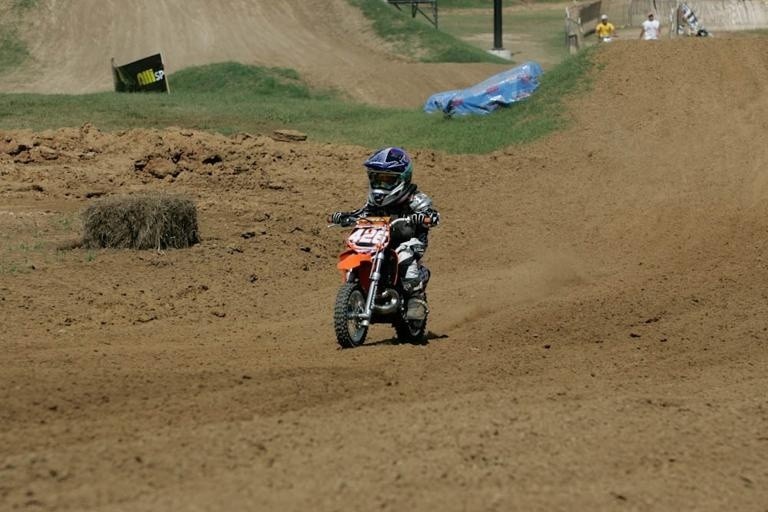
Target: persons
[639,13,661,40]
[335,147,433,330]
[594,14,617,43]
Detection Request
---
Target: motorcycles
[327,210,438,347]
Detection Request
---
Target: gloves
[332,211,347,224]
[406,212,425,225]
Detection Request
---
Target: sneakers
[407,293,427,320]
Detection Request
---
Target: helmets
[365,147,414,208]
